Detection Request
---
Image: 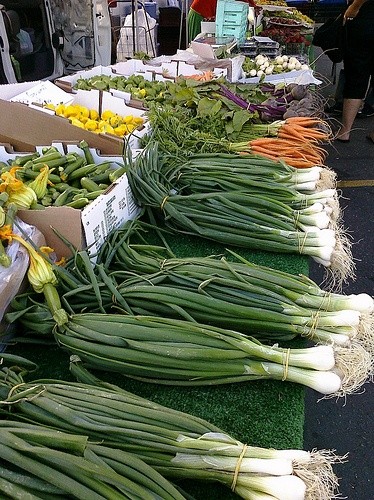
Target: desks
[1,221,314,451]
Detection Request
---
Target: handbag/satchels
[312,13,345,63]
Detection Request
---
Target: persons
[187,0,257,48]
[320,51,374,118]
[331,0,374,144]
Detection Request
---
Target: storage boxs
[2,81,152,261]
[215,0,250,38]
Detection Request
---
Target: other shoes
[334,105,342,112]
[359,107,374,116]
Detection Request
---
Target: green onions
[0,351,348,500]
[84,123,361,295]
[2,209,374,351]
[0,293,374,406]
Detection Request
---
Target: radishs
[242,55,309,78]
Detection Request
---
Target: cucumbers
[0,139,129,211]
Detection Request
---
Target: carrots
[139,101,332,168]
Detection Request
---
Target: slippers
[366,131,374,143]
[331,129,350,142]
